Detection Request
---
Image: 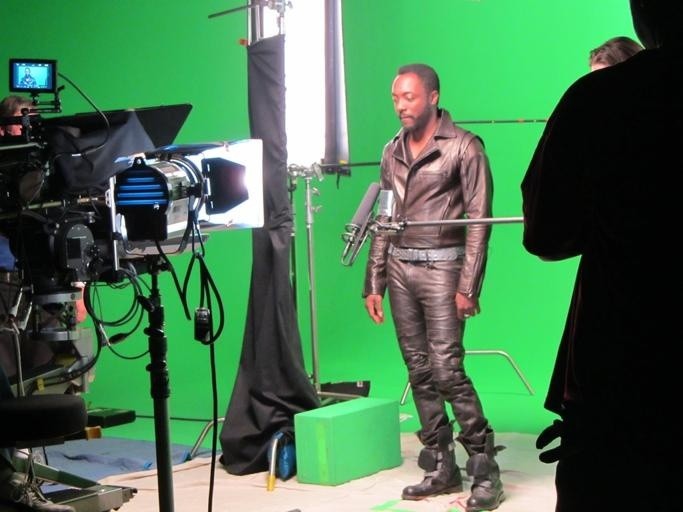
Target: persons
[589,36,643,72]
[362,64,505,512]
[521,0,683,512]
[0,96,88,512]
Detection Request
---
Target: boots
[454,430,507,512]
[400,424,464,501]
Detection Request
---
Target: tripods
[189,179,361,493]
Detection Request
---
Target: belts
[387,241,464,261]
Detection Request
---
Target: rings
[463,314,469,318]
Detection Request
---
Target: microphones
[351,183,381,231]
